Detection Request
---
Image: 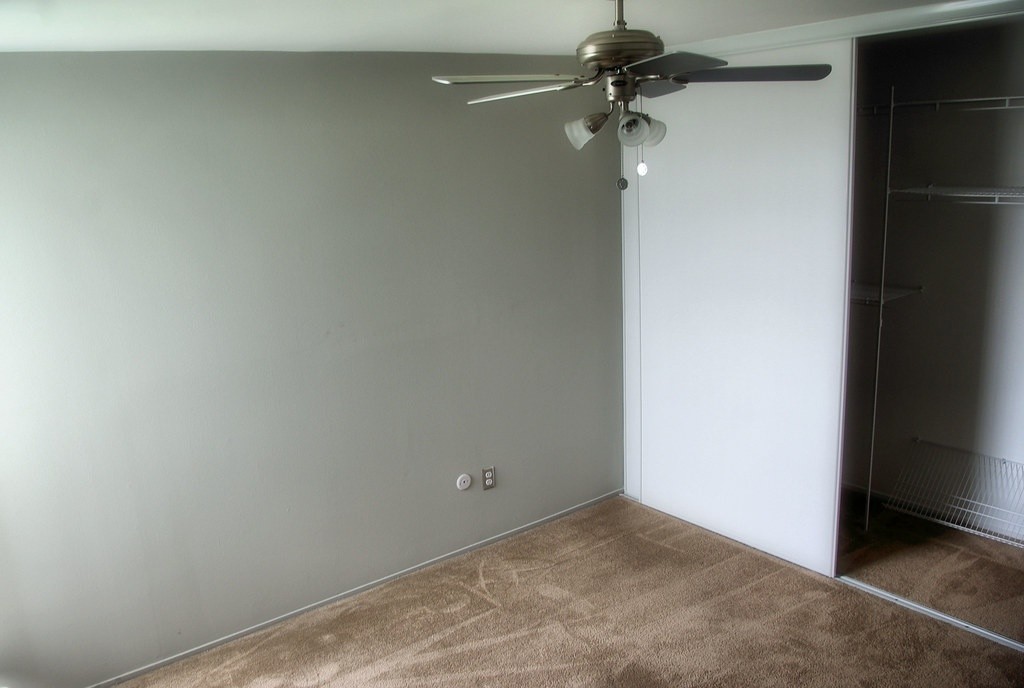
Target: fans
[431,0,832,106]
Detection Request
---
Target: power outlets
[457,474,471,490]
[481,466,496,490]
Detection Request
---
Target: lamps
[564,102,668,152]
